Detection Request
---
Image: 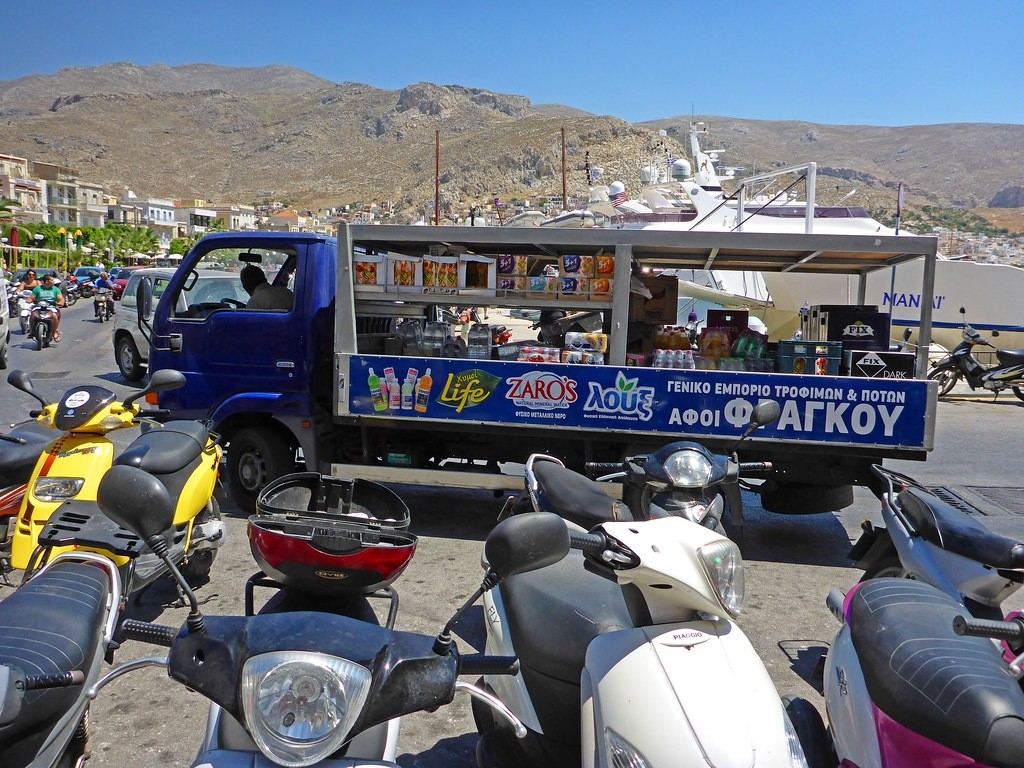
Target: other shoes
[110,309,117,314]
[95,312,99,317]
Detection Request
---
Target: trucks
[136,216,938,516]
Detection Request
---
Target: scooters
[0,494,159,766]
[497,397,783,544]
[7,371,224,633]
[55,283,78,307]
[844,462,1024,654]
[821,574,1024,768]
[1,419,70,589]
[465,489,808,768]
[66,271,100,298]
[13,290,37,335]
[92,290,114,324]
[27,299,63,350]
[927,306,1023,405]
[79,460,576,767]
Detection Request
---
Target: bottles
[395,318,493,361]
[649,322,778,372]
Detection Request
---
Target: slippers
[53,334,60,343]
[27,333,34,340]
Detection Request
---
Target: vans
[112,265,256,382]
[0,268,11,371]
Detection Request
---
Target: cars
[108,266,123,283]
[113,266,150,302]
[12,267,63,290]
[73,266,101,284]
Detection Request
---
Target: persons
[96,260,105,269]
[14,269,43,295]
[26,274,65,343]
[241,265,293,311]
[91,272,116,318]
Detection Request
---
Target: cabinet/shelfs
[351,241,918,379]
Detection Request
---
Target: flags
[608,192,629,207]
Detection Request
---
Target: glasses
[28,273,35,276]
